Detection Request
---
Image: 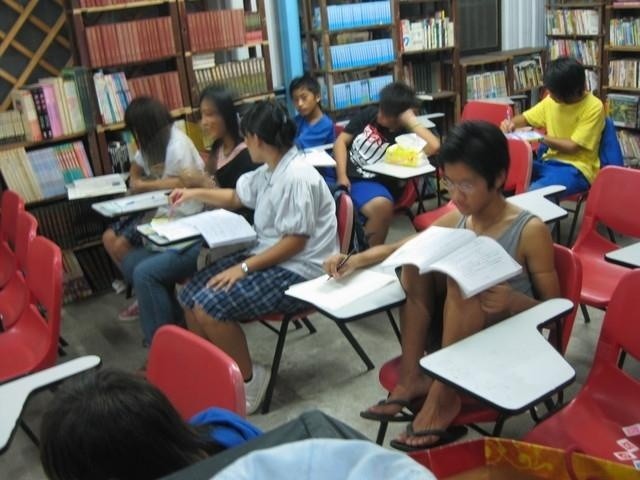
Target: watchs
[241,260,252,275]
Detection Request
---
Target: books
[609,14,640,47]
[466,69,508,102]
[300,37,395,71]
[547,39,599,66]
[28,199,104,250]
[419,102,454,143]
[100,191,168,213]
[547,5,599,35]
[85,16,177,69]
[403,57,455,94]
[0,141,95,209]
[80,0,153,8]
[400,10,454,52]
[301,149,337,167]
[616,130,640,166]
[1,110,25,139]
[607,93,640,129]
[60,245,123,303]
[317,75,393,110]
[284,267,394,313]
[585,69,599,97]
[11,66,95,143]
[608,56,640,89]
[191,111,215,151]
[188,8,263,53]
[191,44,267,103]
[72,176,127,197]
[92,68,183,125]
[504,53,545,93]
[377,225,523,301]
[107,119,187,173]
[503,127,545,144]
[510,95,528,114]
[151,207,257,248]
[311,0,391,31]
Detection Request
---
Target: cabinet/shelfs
[0,128,102,253]
[547,0,640,165]
[292,0,460,201]
[67,0,275,172]
[444,46,550,116]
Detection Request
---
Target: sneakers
[242,363,273,416]
[112,279,127,293]
[118,300,142,321]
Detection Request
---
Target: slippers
[358,394,430,425]
[390,419,469,453]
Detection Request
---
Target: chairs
[414,139,533,235]
[537,116,625,245]
[572,163,640,317]
[0,237,63,451]
[143,324,248,421]
[521,269,640,470]
[240,195,375,416]
[393,175,423,238]
[417,100,514,216]
[375,244,584,437]
[0,188,22,288]
[333,124,346,136]
[2,211,41,327]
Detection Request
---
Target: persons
[178,97,341,415]
[324,81,440,249]
[499,56,606,205]
[360,119,565,452]
[290,76,335,171]
[38,366,262,480]
[117,84,264,346]
[103,96,206,295]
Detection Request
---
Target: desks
[69,172,130,197]
[136,210,245,245]
[363,159,435,180]
[92,188,173,217]
[506,183,569,224]
[0,348,102,458]
[605,236,640,268]
[418,298,577,440]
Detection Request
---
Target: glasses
[439,177,475,194]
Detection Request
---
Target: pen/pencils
[506,109,511,133]
[126,194,154,205]
[326,246,358,282]
[168,187,185,220]
